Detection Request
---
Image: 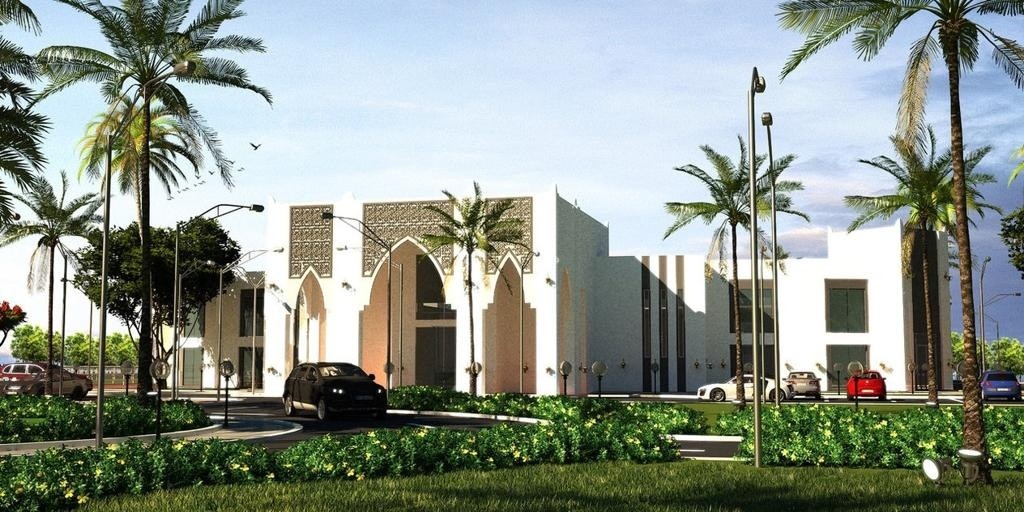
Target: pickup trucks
[782,372,821,400]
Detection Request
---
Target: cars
[696,374,791,402]
[979,370,1021,401]
[0,363,93,401]
[845,372,886,401]
[279,362,387,422]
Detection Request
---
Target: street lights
[320,212,403,401]
[96,61,198,447]
[520,251,541,396]
[170,204,265,404]
[980,256,1022,373]
[216,247,285,405]
[749,66,780,468]
